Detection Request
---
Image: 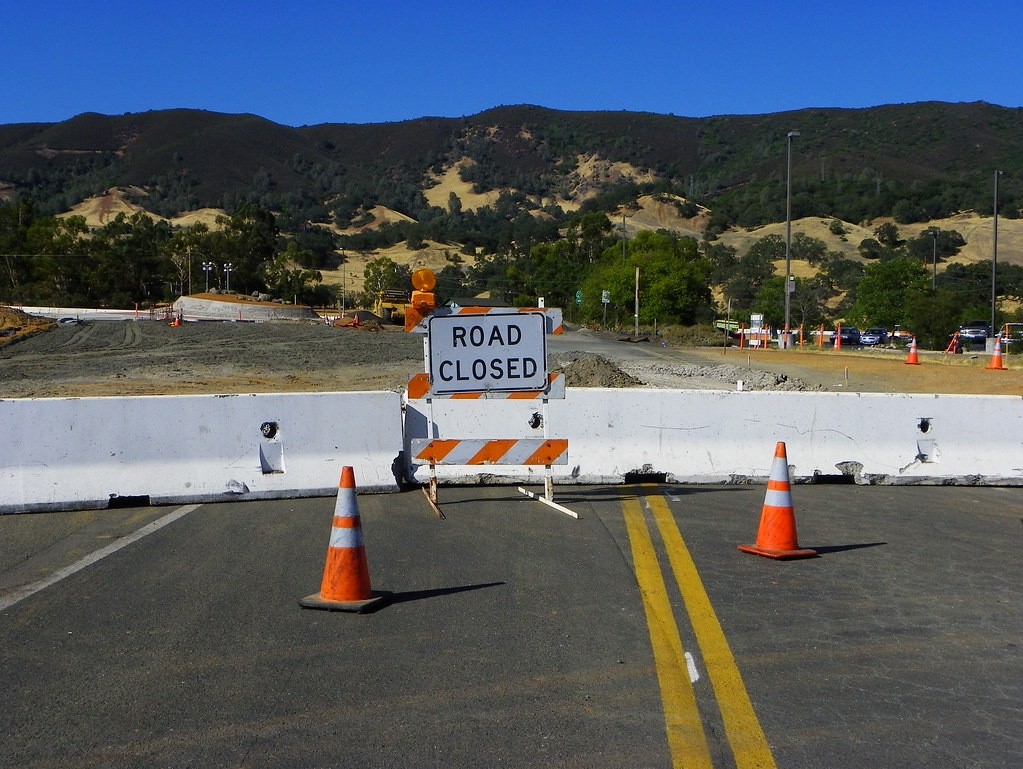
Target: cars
[899,335,912,343]
[829,327,860,345]
[889,336,899,343]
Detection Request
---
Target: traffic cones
[173,310,181,326]
[985,336,1008,370]
[904,336,921,365]
[354,313,359,326]
[832,336,839,351]
[735,441,820,556]
[300,465,394,615]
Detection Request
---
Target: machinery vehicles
[374,288,412,322]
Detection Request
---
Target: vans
[861,328,887,345]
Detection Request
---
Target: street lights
[623,214,633,262]
[991,169,1005,337]
[202,261,213,292]
[340,247,345,317]
[929,230,942,289]
[785,131,801,332]
[223,262,233,290]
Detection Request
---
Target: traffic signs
[429,312,548,394]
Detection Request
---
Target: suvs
[959,320,996,343]
[995,323,1023,344]
